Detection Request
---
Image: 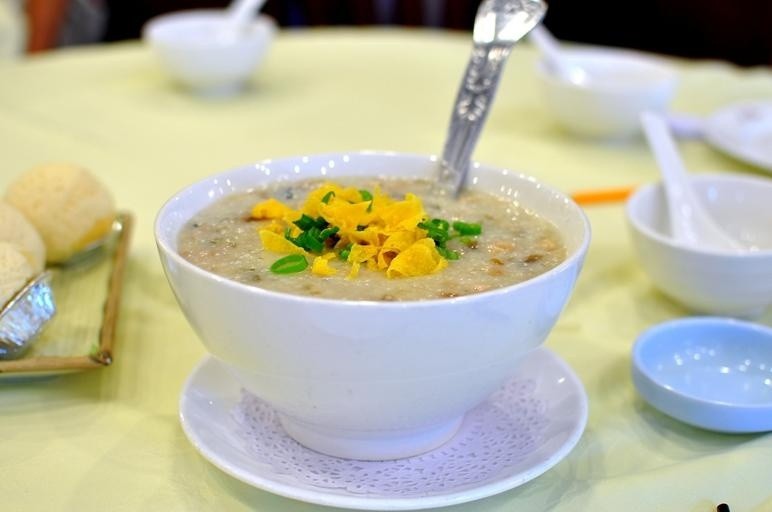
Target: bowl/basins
[535,46,678,143]
[150,147,592,463]
[142,9,277,102]
[623,173,772,319]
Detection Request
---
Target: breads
[0,161,116,317]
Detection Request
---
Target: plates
[176,346,590,511]
[632,316,771,433]
[704,100,772,174]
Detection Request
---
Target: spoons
[637,109,744,255]
